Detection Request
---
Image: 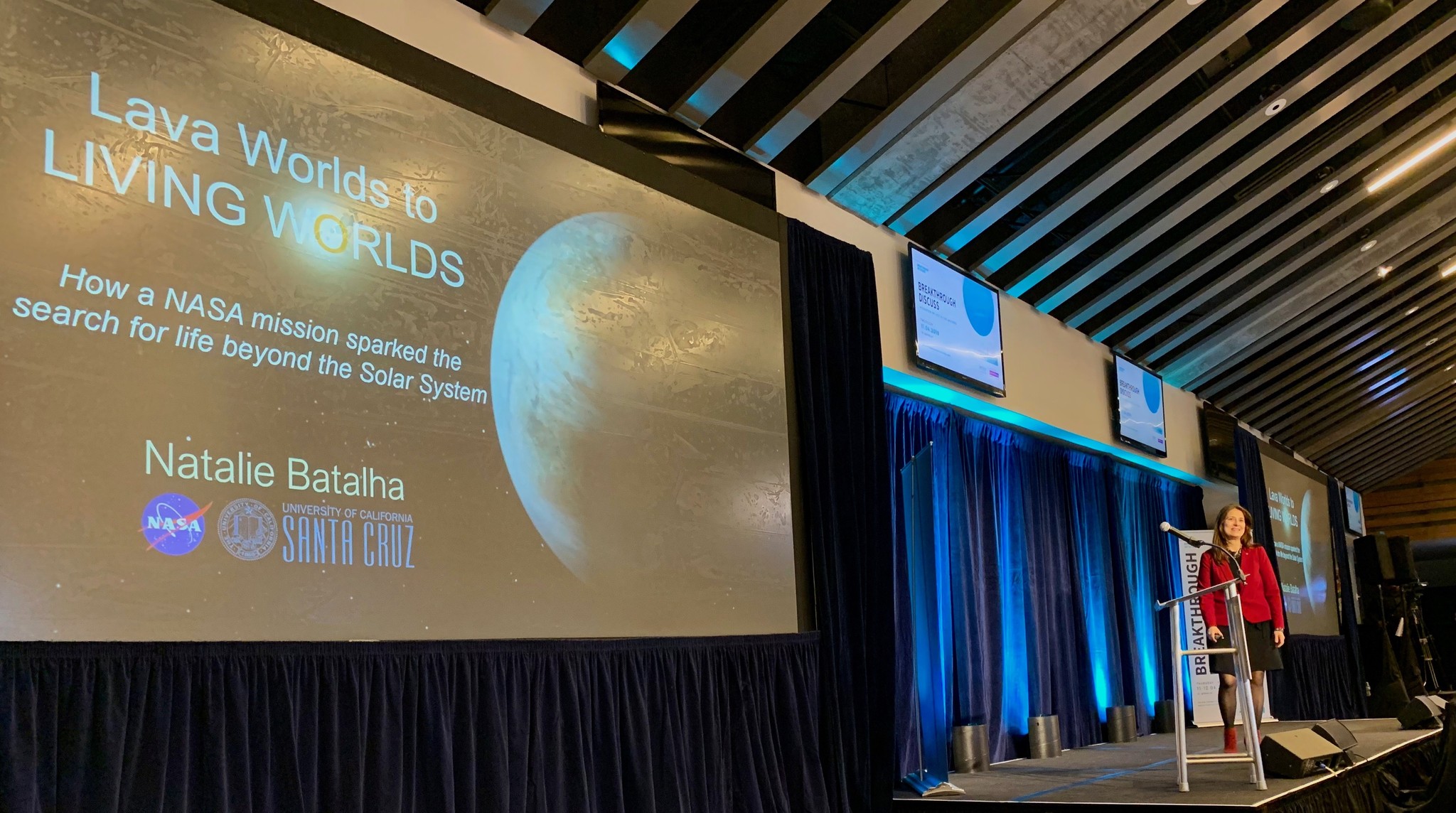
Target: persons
[1195,504,1286,753]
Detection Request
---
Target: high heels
[1244,729,1261,751]
[1224,727,1238,753]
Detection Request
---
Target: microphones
[1160,522,1201,549]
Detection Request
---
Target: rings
[1207,631,1211,635]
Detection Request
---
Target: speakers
[1259,718,1358,778]
[1353,533,1418,583]
[1397,695,1449,730]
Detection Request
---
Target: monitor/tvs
[1113,350,1167,459]
[907,241,1006,399]
[596,79,777,211]
[1203,400,1238,486]
[1344,484,1363,537]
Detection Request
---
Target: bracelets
[1275,628,1284,633]
